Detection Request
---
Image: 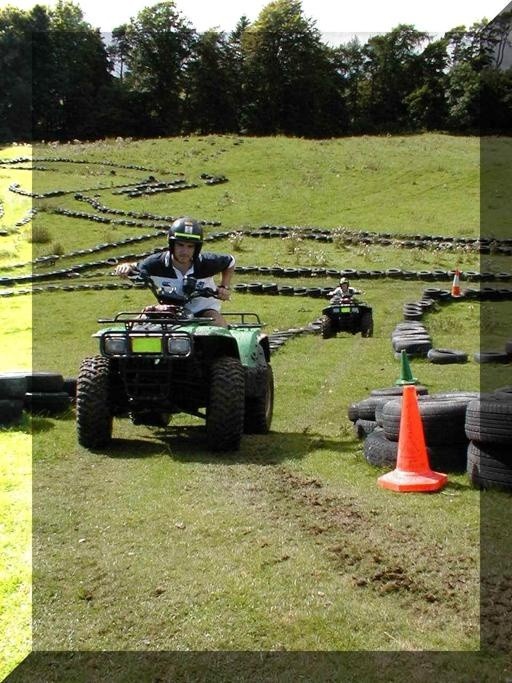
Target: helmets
[340,277,349,289]
[168,217,203,256]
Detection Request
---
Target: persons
[328,277,363,303]
[114,217,236,328]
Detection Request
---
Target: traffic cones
[451,268,461,298]
[396,350,419,384]
[379,386,450,491]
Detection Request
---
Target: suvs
[76,270,274,451]
[321,290,375,341]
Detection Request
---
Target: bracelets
[218,285,229,289]
